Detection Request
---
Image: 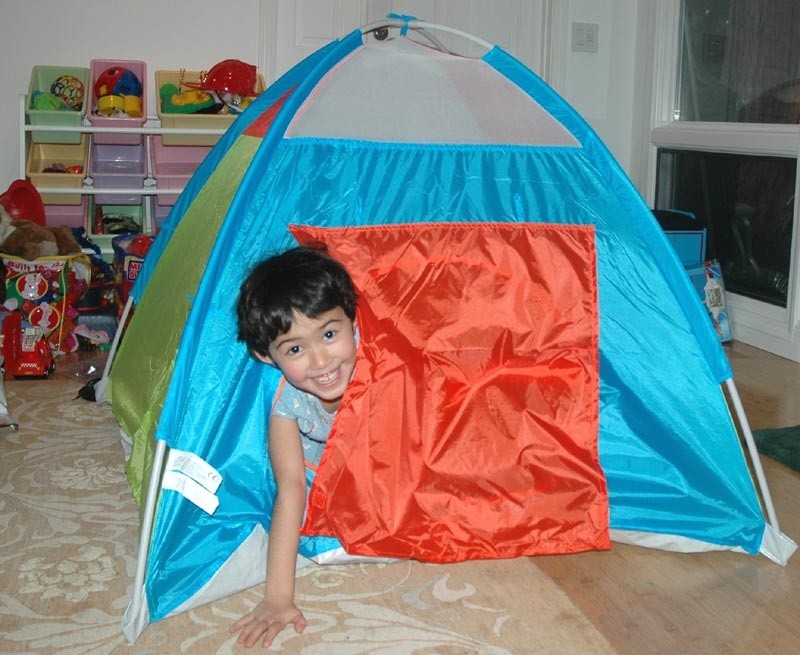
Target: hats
[184,58,259,95]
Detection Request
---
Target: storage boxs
[26,60,266,262]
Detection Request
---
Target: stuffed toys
[0,216,81,257]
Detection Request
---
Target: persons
[230,246,357,648]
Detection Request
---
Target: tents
[88,12,797,645]
[725,77,800,253]
